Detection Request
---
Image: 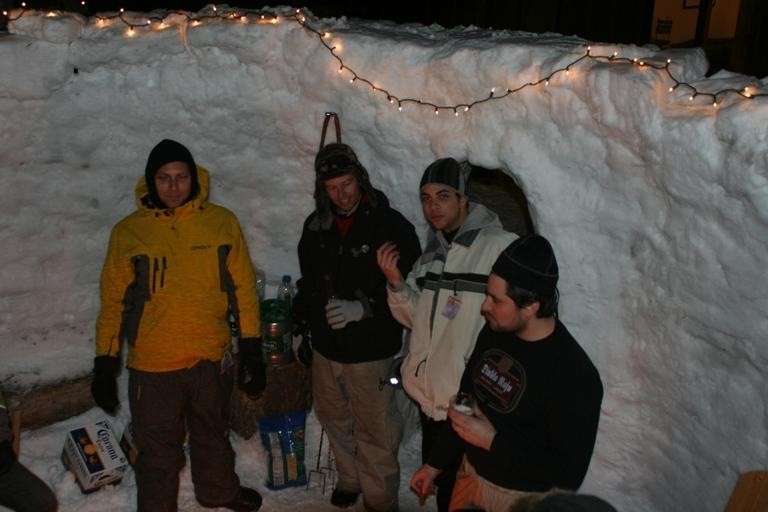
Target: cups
[450,393,477,416]
[259,299,293,364]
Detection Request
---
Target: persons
[507,488,617,512]
[376,157,521,512]
[410,233,604,512]
[90,139,266,512]
[286,142,422,512]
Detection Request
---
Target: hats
[312,142,381,224]
[143,138,202,209]
[417,156,467,198]
[491,233,560,297]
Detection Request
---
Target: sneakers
[194,484,263,512]
[330,487,362,509]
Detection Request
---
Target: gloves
[89,354,122,414]
[324,297,365,331]
[237,336,269,400]
[290,319,316,369]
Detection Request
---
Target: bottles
[277,274,294,302]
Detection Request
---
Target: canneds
[261,310,292,365]
